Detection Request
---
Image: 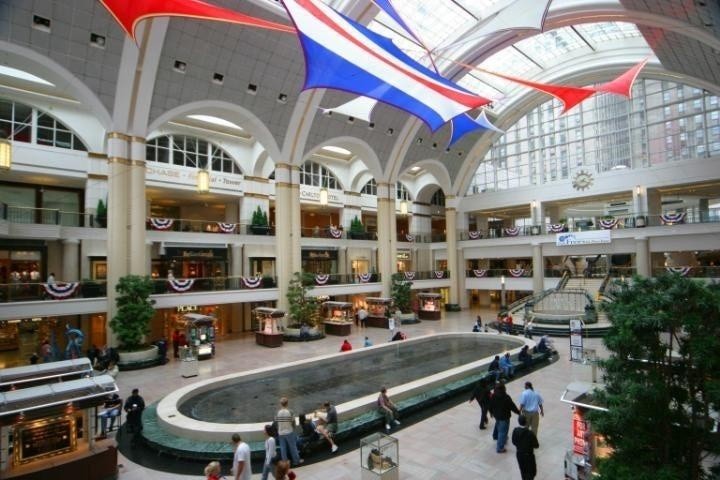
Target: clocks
[571,169,595,193]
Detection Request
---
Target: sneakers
[386,424,391,429]
[331,444,338,453]
[394,420,400,424]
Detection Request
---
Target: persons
[230,433,253,480]
[29,352,40,365]
[204,460,226,480]
[41,272,57,300]
[470,335,558,480]
[124,387,145,431]
[469,306,538,343]
[260,396,340,479]
[364,335,372,346]
[376,383,401,434]
[340,339,352,352]
[86,344,113,372]
[98,392,122,433]
[358,306,369,328]
[40,339,52,363]
[171,327,182,359]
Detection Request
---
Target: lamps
[198,170,210,194]
[0,138,12,173]
[320,189,328,205]
[401,200,407,215]
[636,184,640,195]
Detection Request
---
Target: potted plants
[390,272,415,320]
[349,215,367,239]
[369,266,379,282]
[96,198,107,228]
[82,278,101,298]
[262,272,273,287]
[249,205,270,235]
[108,275,158,363]
[284,271,322,335]
